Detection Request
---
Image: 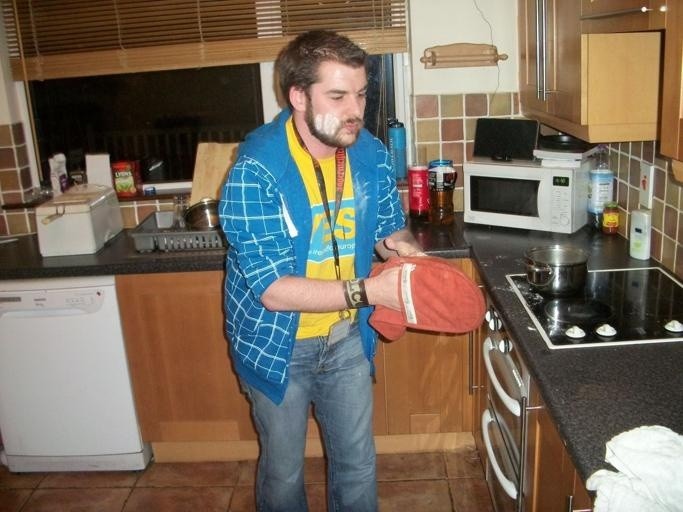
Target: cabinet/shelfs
[373,257,477,455]
[472,258,593,512]
[513,1,666,144]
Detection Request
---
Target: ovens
[474,292,534,512]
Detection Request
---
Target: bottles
[586,143,614,230]
[388,122,407,180]
[428,158,457,226]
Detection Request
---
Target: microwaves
[462,151,602,235]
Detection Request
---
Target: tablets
[472,117,540,163]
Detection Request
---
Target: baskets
[128,209,225,253]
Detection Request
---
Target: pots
[522,242,592,295]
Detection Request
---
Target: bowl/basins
[182,197,221,231]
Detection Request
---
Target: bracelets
[343,278,370,309]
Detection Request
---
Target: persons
[218,30,428,512]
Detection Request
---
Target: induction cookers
[507,266,683,350]
